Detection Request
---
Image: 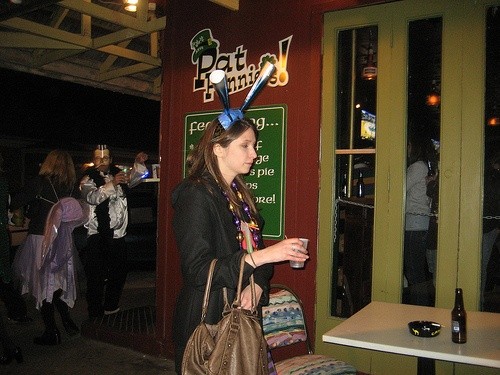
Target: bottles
[356,169,365,198]
[427,159,434,185]
[451,288,467,344]
[341,171,347,197]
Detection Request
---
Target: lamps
[486,73,500,126]
[123,0,138,11]
[362,27,377,80]
[425,72,440,107]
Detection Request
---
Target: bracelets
[249,253,257,267]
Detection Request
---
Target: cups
[289,238,308,268]
[152,164,160,178]
[121,168,131,183]
[14,208,24,226]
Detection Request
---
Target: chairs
[261,284,356,375]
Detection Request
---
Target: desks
[9,225,28,246]
[322,302,500,375]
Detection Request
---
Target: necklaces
[216,180,259,253]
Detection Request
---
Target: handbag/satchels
[182,256,270,375]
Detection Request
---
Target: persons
[79,147,148,315]
[13,150,88,345]
[404,135,500,307]
[0,153,23,365]
[171,66,309,375]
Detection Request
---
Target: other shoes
[63,327,80,338]
[35,328,62,345]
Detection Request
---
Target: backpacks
[37,178,84,223]
